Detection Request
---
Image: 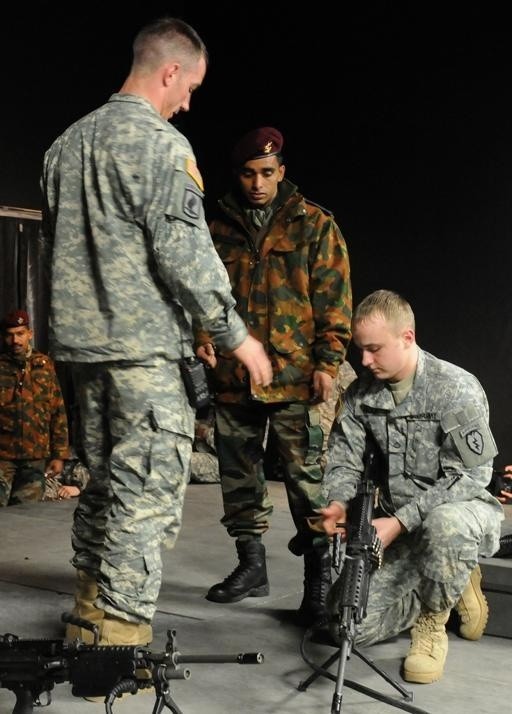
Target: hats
[1,310,28,330]
[227,126,284,169]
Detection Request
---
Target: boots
[84,609,155,704]
[63,569,152,647]
[207,540,269,603]
[454,564,489,640]
[402,607,451,684]
[295,541,333,627]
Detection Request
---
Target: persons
[494,460,512,507]
[0,310,81,506]
[318,288,504,686]
[189,125,354,626]
[37,20,275,655]
[190,403,220,484]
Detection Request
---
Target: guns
[1,611,264,710]
[295,442,412,710]
